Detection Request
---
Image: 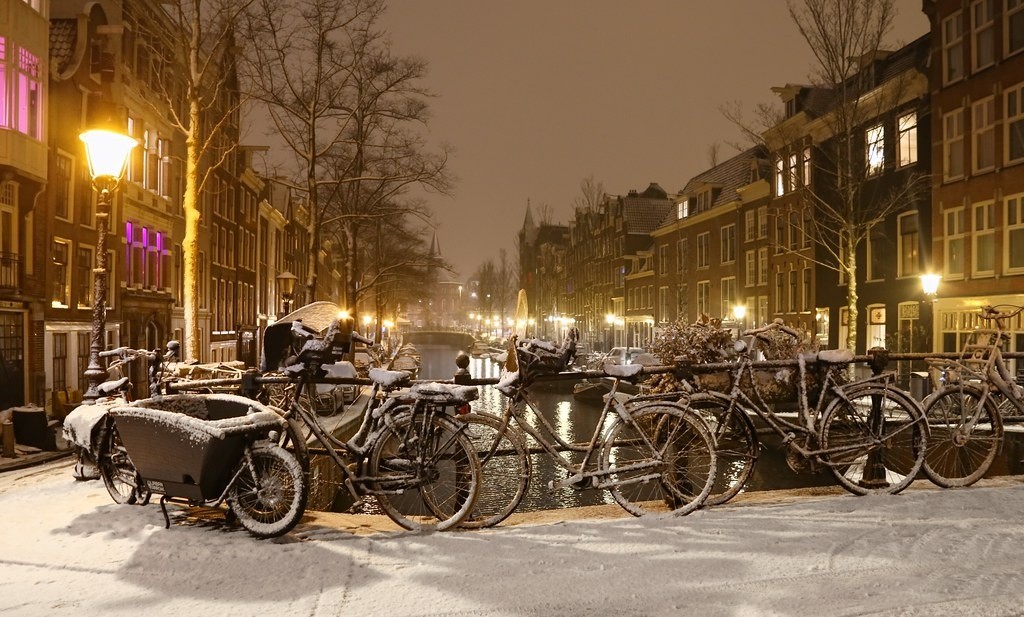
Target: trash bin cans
[909,371,930,403]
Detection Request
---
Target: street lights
[76,104,143,404]
[276,268,298,314]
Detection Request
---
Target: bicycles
[87,296,1022,544]
